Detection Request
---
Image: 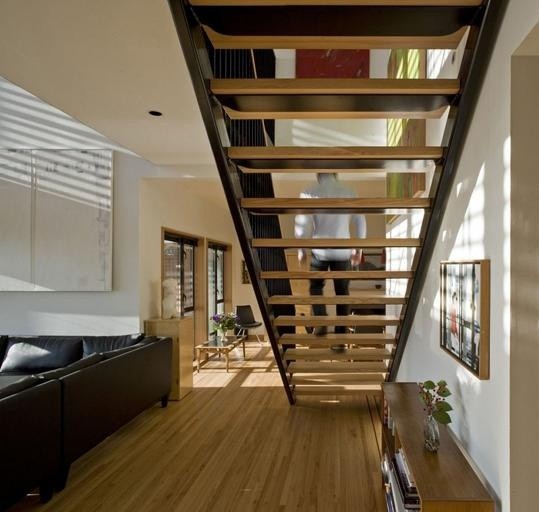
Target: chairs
[234,305,262,346]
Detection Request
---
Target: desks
[194,335,248,373]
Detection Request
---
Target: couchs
[0,334,173,511]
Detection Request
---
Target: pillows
[82,331,146,359]
[0,336,84,374]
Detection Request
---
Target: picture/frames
[439,259,491,380]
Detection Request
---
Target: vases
[423,414,441,456]
[219,326,228,342]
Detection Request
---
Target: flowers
[417,377,454,425]
[208,312,241,336]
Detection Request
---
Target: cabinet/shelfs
[143,315,196,401]
[380,382,496,512]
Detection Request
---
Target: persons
[292,172,366,352]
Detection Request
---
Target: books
[383,393,420,512]
